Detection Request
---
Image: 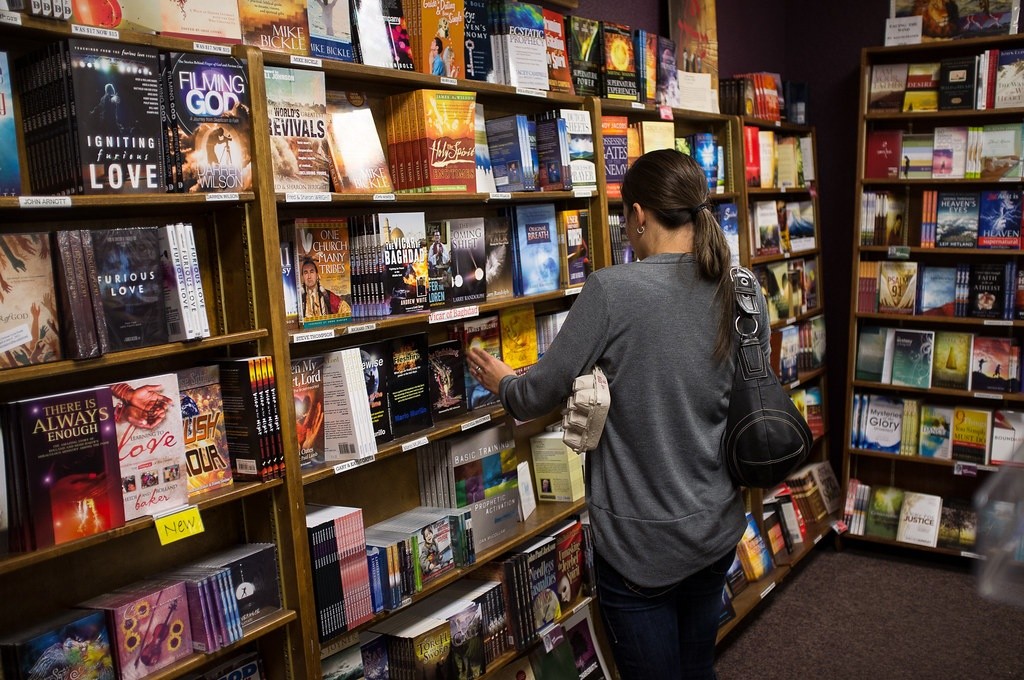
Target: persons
[429,37,445,76]
[443,48,460,78]
[509,162,518,175]
[548,164,558,182]
[465,149,772,679]
[417,278,426,295]
[428,231,450,264]
[420,526,443,574]
[542,480,550,492]
[302,262,351,316]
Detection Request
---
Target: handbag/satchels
[724,266,814,488]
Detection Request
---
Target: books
[1,1,1024,680]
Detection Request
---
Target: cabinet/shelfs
[837,31,1024,562]
[0,7,836,680]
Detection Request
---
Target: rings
[476,367,481,371]
